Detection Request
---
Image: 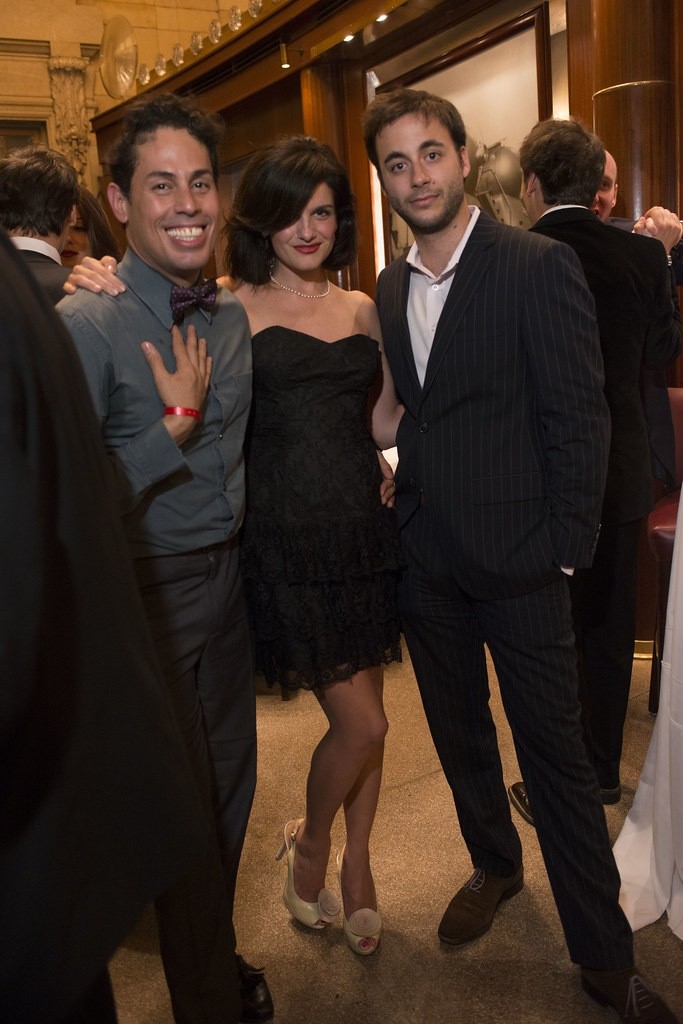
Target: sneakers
[438,863,524,945]
[581,964,680,1024]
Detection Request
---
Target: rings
[392,481,396,488]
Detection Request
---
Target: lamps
[280,43,304,69]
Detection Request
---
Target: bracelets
[667,254,673,266]
[163,406,202,422]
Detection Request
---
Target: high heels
[336,842,382,956]
[275,818,341,930]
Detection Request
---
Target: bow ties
[170,275,217,321]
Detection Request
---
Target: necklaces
[269,263,331,297]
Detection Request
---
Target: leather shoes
[508,781,535,827]
[235,953,274,1020]
[600,784,622,805]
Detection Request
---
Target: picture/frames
[374,0,553,267]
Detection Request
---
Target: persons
[0,139,210,1024]
[59,134,410,956]
[52,93,274,1024]
[509,122,683,824]
[213,87,681,1024]
